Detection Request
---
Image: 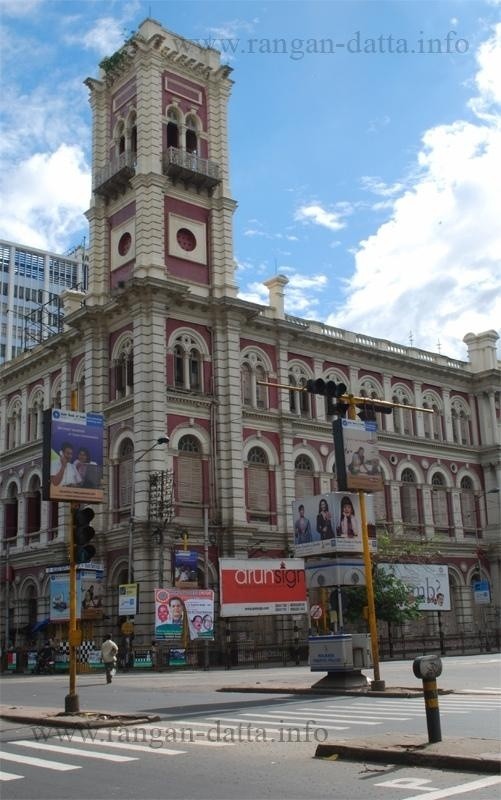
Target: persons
[348,446,369,475]
[432,593,444,607]
[38,639,52,671]
[82,585,97,609]
[99,634,119,685]
[336,495,359,538]
[157,596,213,640]
[73,446,104,487]
[294,504,312,544]
[316,499,335,540]
[50,443,82,488]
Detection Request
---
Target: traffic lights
[74,506,97,564]
[306,377,348,399]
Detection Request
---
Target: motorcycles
[34,653,56,674]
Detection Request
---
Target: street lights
[126,435,169,667]
[475,487,500,651]
